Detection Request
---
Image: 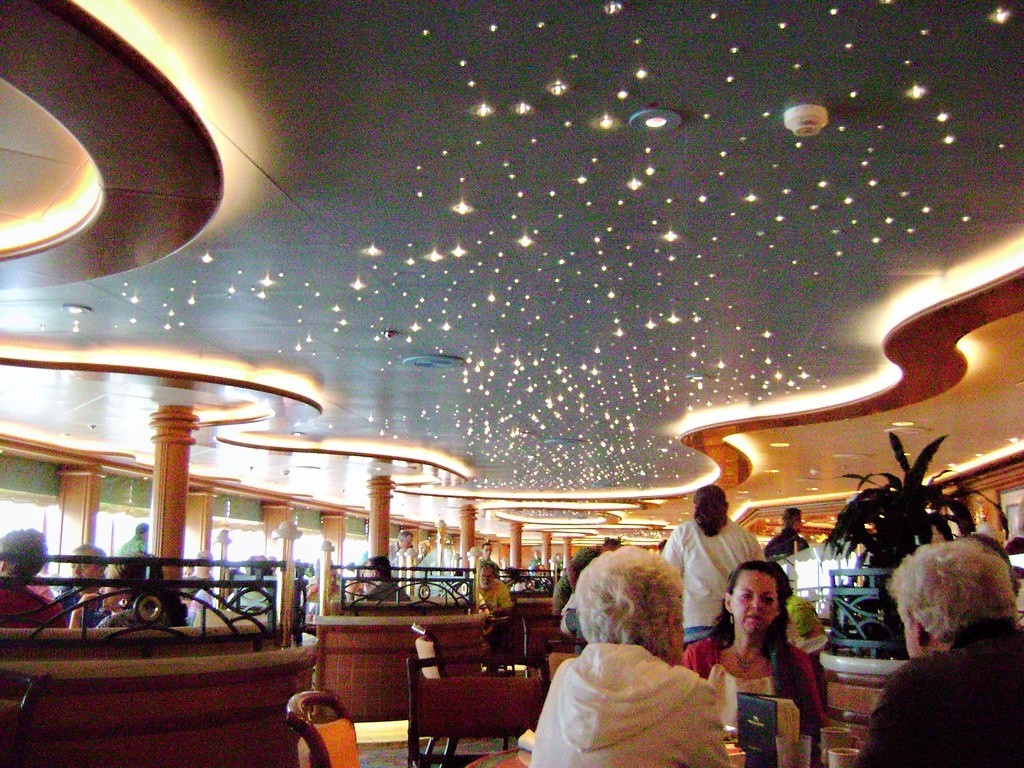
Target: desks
[465,735,829,768]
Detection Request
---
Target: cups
[819,727,861,764]
[776,732,811,768]
[826,748,861,768]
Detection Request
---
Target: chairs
[408,654,553,768]
[286,691,361,768]
[541,637,588,700]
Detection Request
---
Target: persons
[681,559,828,768]
[764,508,810,560]
[661,485,766,648]
[528,545,731,768]
[856,541,1024,768]
[0,521,621,655]
